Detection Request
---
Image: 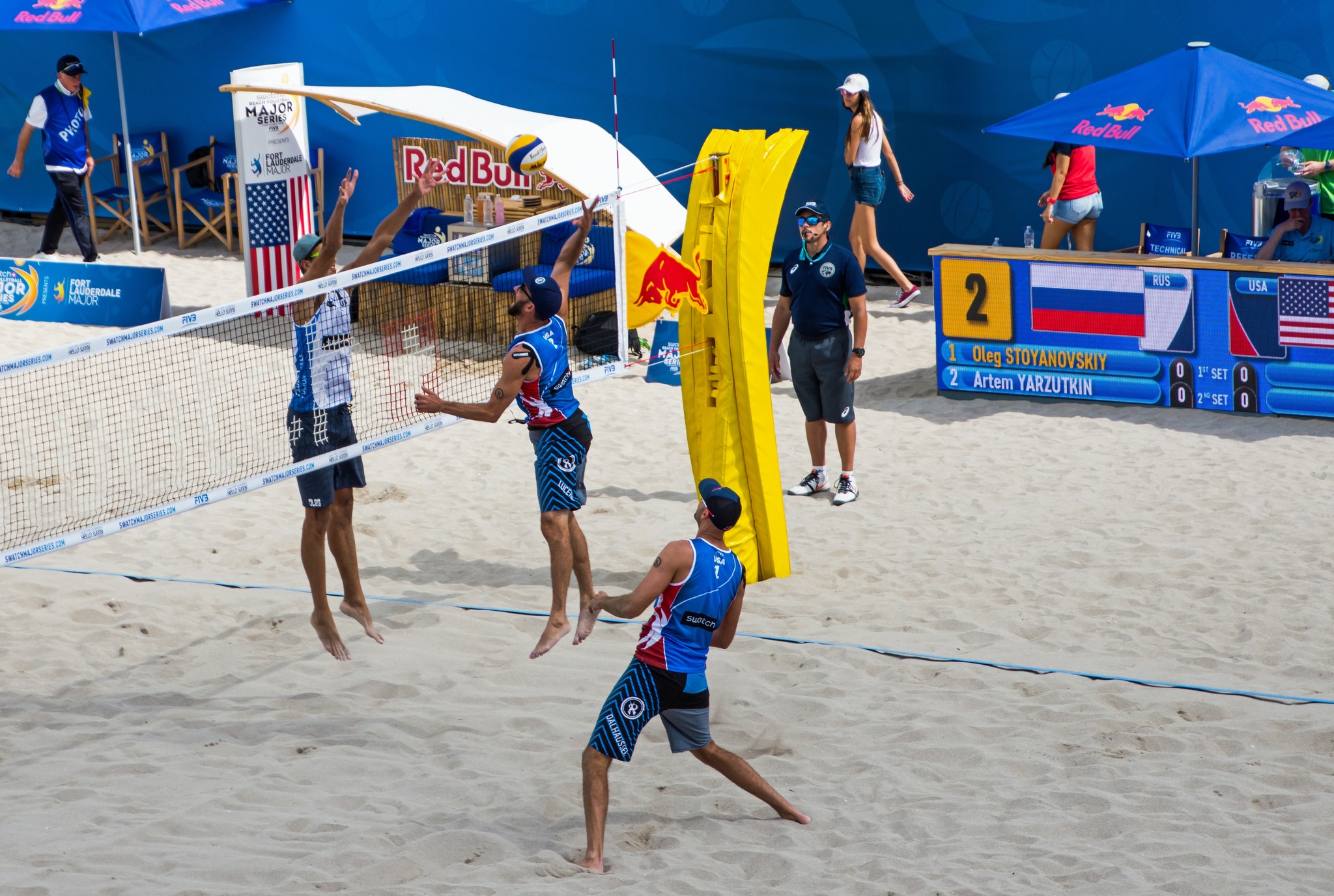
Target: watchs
[852,347,865,357]
[1325,161,1332,172]
[1046,197,1056,203]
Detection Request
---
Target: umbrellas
[981,41,1334,257]
[0,0,292,255]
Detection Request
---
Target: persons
[1037,93,1104,252]
[1279,74,1334,221]
[413,196,603,661]
[1254,180,1334,264]
[284,155,448,663]
[571,478,810,873]
[835,72,921,309]
[7,54,102,264]
[768,200,867,505]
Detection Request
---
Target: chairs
[172,136,238,251]
[233,147,324,254]
[1205,228,1280,262]
[373,213,464,284]
[84,131,177,246]
[492,221,615,297]
[1110,222,1200,257]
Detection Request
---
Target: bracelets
[895,180,904,185]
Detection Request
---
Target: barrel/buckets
[1251,178,1321,237]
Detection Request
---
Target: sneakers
[890,284,921,308]
[833,474,859,505]
[788,468,830,496]
[83,255,108,264]
[36,249,60,261]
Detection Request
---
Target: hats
[1284,181,1311,211]
[836,73,869,93]
[293,233,323,269]
[522,264,562,319]
[698,478,742,531]
[795,199,831,219]
[56,54,88,76]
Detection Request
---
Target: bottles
[483,196,493,225]
[476,192,485,222]
[992,237,1002,246]
[494,193,505,223]
[1024,226,1034,248]
[463,194,473,225]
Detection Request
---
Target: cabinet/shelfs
[447,220,520,286]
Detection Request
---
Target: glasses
[517,281,535,306]
[840,89,855,97]
[798,216,827,227]
